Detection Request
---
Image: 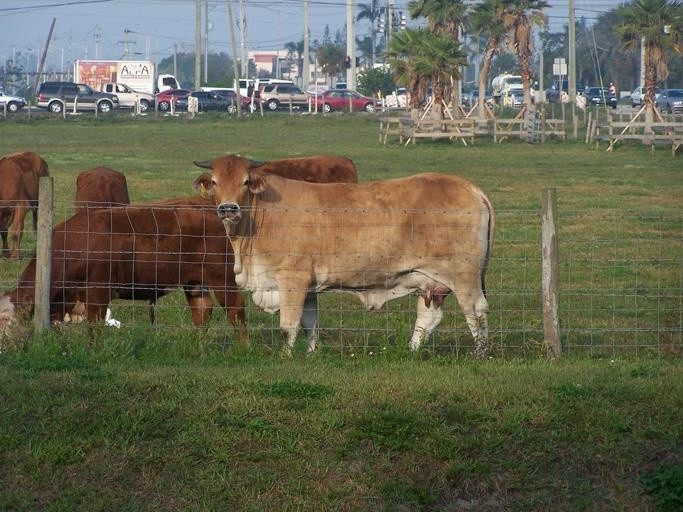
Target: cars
[154,87,263,114]
[394,85,493,107]
[306,81,384,113]
[546,78,682,114]
[0,91,26,114]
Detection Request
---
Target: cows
[192,157,496,362]
[0,150,51,260]
[0,197,252,353]
[74,166,129,212]
[250,157,357,187]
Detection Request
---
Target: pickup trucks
[102,82,155,112]
[200,79,257,97]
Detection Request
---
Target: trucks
[72,57,182,95]
[491,74,532,108]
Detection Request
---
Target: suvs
[254,77,317,111]
[35,81,121,114]
